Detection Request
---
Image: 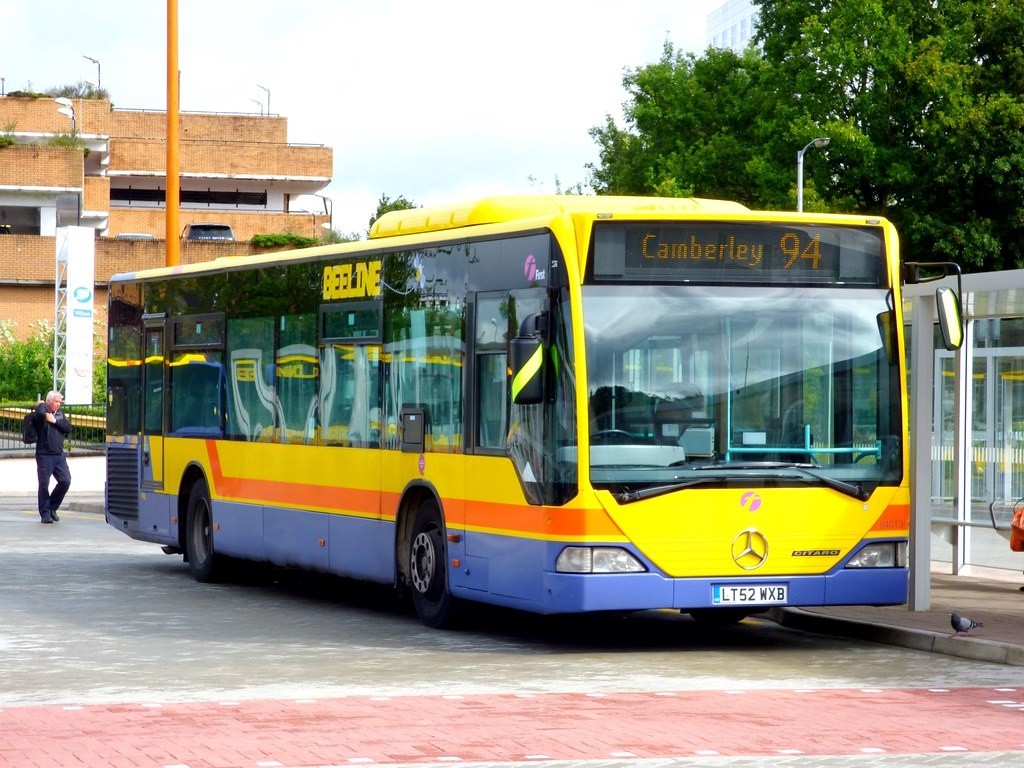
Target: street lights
[55,95,81,145]
[795,134,833,212]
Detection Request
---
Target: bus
[104,193,965,631]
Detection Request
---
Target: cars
[115,233,157,239]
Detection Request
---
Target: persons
[508,405,569,504]
[34,391,72,524]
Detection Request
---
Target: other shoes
[41,513,54,523]
[51,509,59,521]
[1019,586,1024,591]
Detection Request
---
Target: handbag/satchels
[23,413,38,444]
[1010,509,1024,552]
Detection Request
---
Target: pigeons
[949,610,984,639]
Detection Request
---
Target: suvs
[180,223,237,241]
